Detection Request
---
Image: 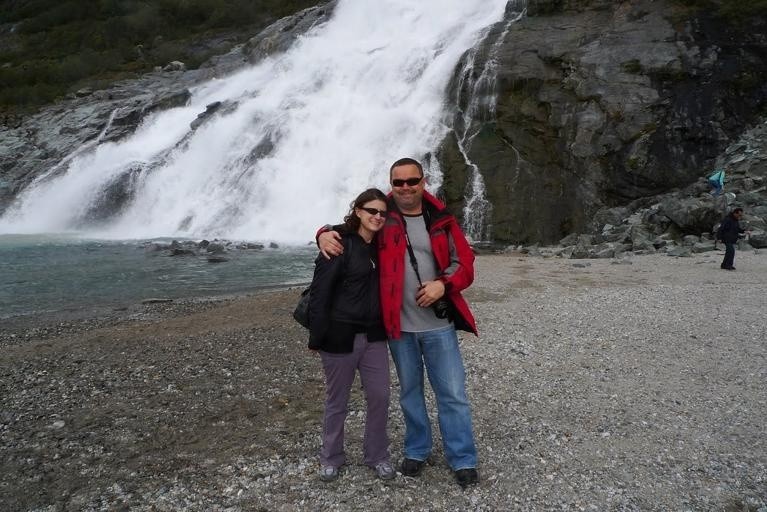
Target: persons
[716,208,752,271]
[314,156,482,486]
[308,187,398,482]
[708,169,731,195]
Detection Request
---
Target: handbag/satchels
[293,283,312,329]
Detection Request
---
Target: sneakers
[376,457,427,479]
[456,468,477,480]
[320,465,338,480]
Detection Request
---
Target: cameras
[417,294,450,319]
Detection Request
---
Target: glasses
[392,177,423,186]
[359,208,388,217]
